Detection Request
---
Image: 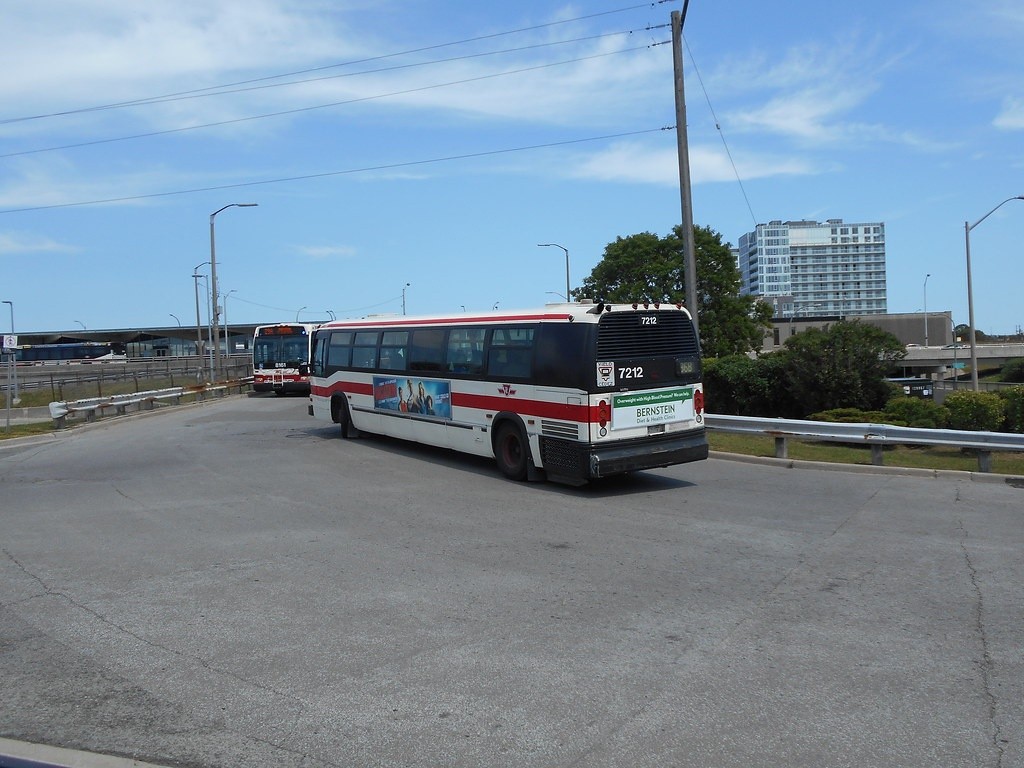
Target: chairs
[375,341,531,370]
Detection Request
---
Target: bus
[308,299,709,482]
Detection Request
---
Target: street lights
[191,273,217,384]
[545,291,567,302]
[538,243,570,303]
[169,314,185,356]
[209,202,259,377]
[787,303,823,337]
[924,274,932,346]
[74,319,86,331]
[195,261,221,371]
[402,283,411,316]
[962,197,1024,391]
[932,311,958,381]
[223,289,237,358]
[1,300,17,398]
